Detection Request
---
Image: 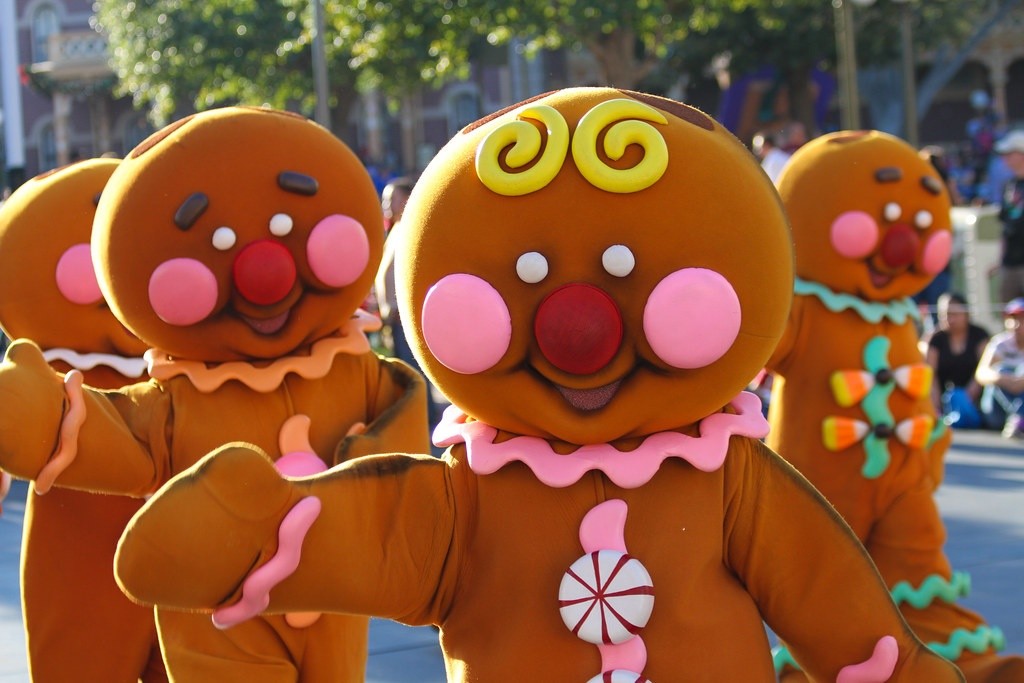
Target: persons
[974,298,1024,437]
[971,131,1014,207]
[758,121,807,184]
[919,143,976,206]
[992,131,1024,304]
[374,178,419,370]
[919,291,992,430]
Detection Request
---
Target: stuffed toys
[0,156,168,683]
[0,106,430,683]
[761,129,1024,683]
[113,87,967,683]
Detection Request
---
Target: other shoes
[1002,413,1022,438]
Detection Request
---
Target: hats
[993,132,1024,155]
[1004,297,1024,315]
